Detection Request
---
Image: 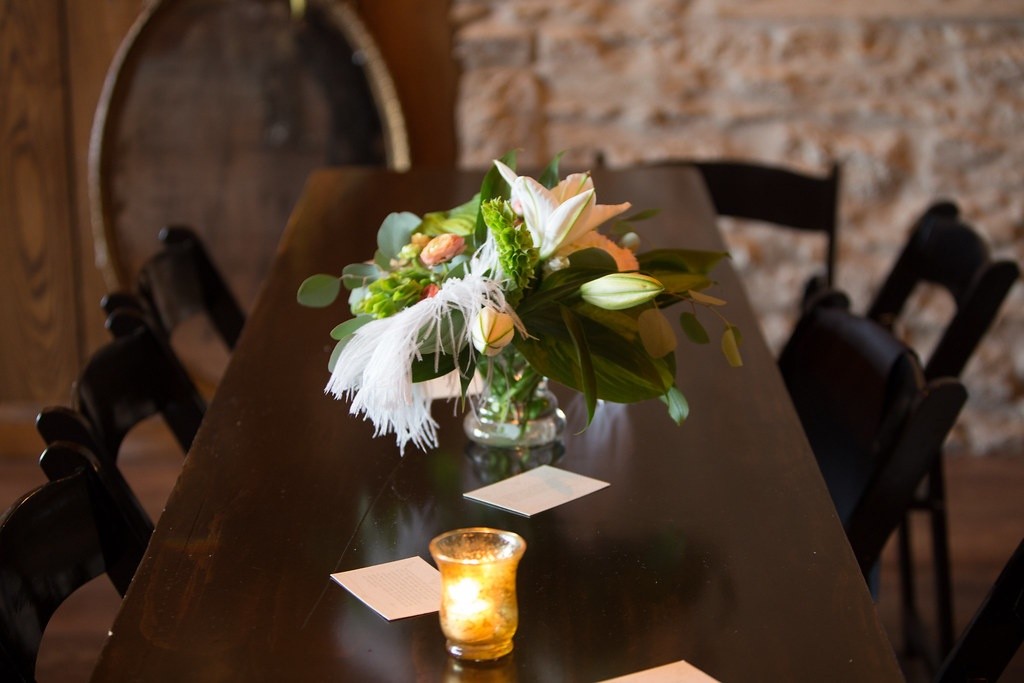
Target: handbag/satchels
[778,277,969,598]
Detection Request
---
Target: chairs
[0,219,250,683]
[696,159,1024,683]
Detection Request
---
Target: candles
[428,528,526,661]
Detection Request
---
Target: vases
[464,362,566,450]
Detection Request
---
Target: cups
[428,527,526,660]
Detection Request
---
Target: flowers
[293,148,742,452]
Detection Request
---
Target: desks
[92,163,908,683]
